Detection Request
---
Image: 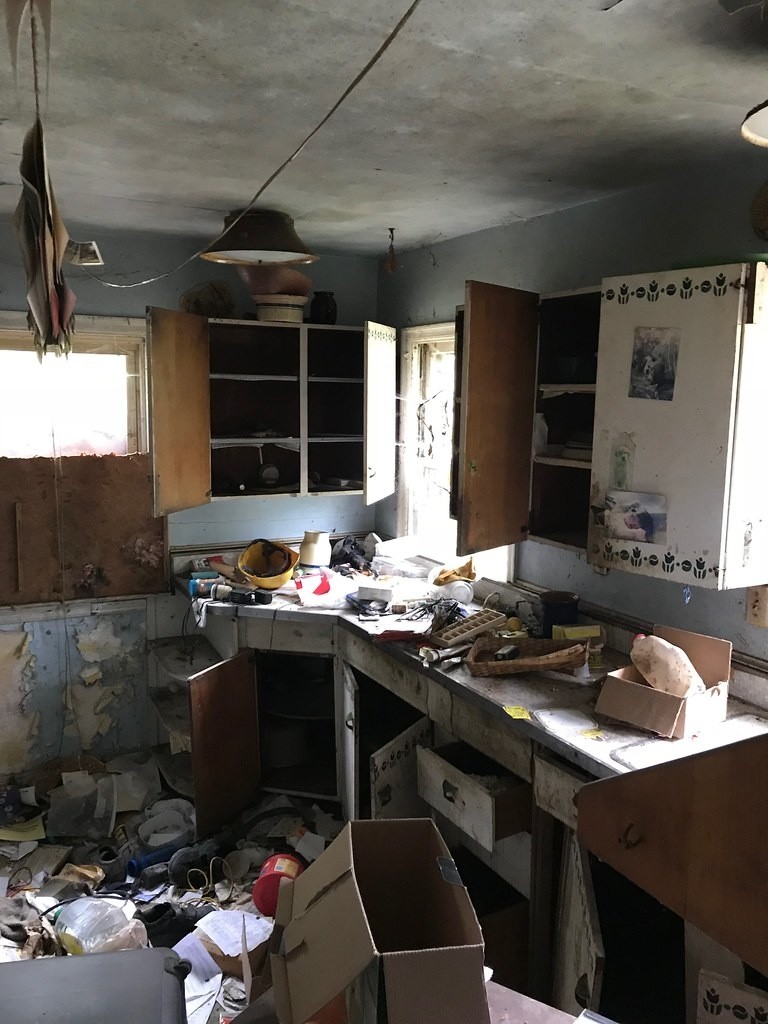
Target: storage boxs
[594,622,734,740]
[276,818,492,1024]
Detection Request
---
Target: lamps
[199,208,321,266]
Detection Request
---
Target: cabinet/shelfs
[449,263,768,590]
[146,306,398,518]
[148,592,339,844]
[529,742,768,1024]
[335,620,527,995]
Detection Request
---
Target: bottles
[311,291,337,325]
[300,533,331,577]
[54,896,130,954]
[629,634,704,695]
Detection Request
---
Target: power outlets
[744,587,768,629]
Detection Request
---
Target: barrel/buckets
[253,854,304,916]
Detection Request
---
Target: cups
[539,592,580,636]
